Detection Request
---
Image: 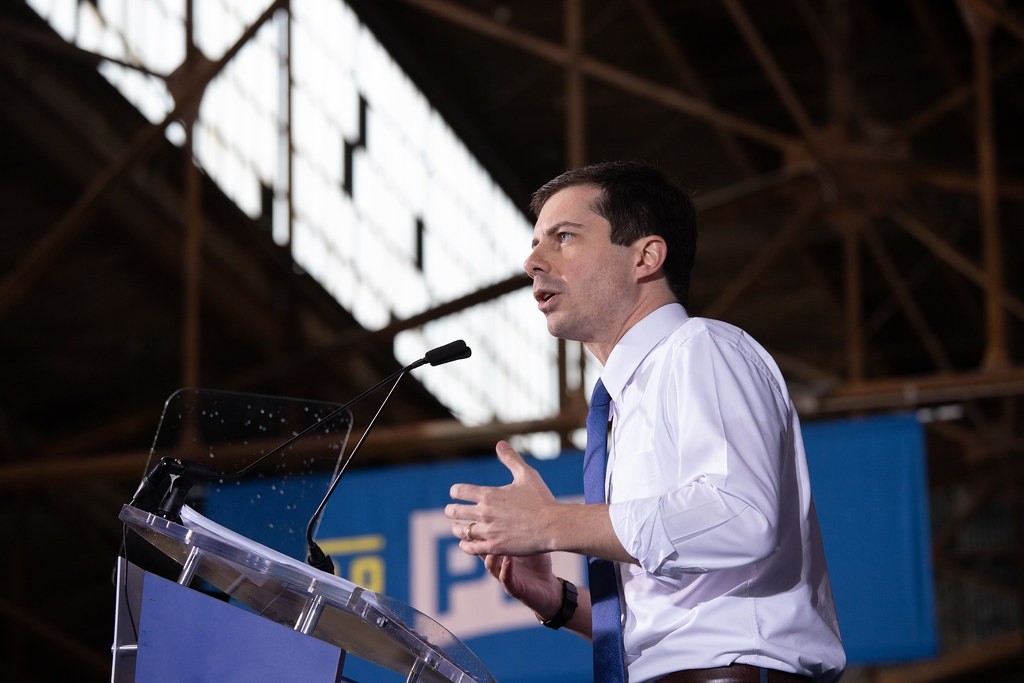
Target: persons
[445,159,845,683]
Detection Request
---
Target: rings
[467,523,476,540]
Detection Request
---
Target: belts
[646,663,821,682]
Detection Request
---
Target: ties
[582,375,627,683]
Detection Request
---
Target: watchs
[535,577,578,630]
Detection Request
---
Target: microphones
[130,340,472,577]
